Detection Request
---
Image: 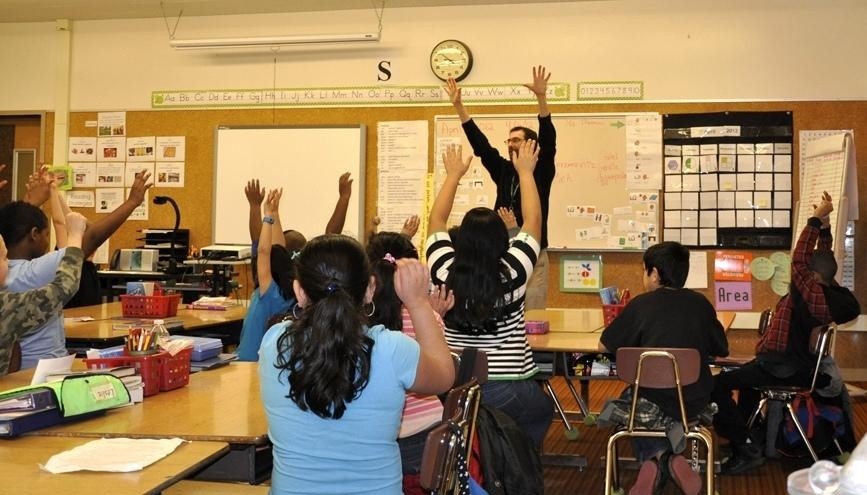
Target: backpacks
[478,404,547,495]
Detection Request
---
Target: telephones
[110,249,121,271]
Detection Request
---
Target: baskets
[120,293,182,318]
[603,304,626,328]
[82,338,194,397]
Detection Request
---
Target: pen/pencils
[186,304,226,311]
[124,322,158,352]
[609,288,630,306]
[790,134,852,286]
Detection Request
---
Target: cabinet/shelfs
[136,228,188,266]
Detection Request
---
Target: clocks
[428,39,474,83]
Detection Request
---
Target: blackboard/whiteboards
[433,112,663,253]
[212,124,367,251]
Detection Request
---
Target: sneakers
[723,441,768,475]
[629,457,664,495]
[669,455,702,495]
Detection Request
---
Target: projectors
[199,245,252,261]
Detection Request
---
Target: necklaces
[359,231,455,475]
[0,162,153,372]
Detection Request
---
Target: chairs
[743,327,839,470]
[603,348,721,494]
[402,344,491,494]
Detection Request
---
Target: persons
[244,170,352,296]
[226,186,299,362]
[442,65,555,314]
[400,215,422,241]
[596,242,729,495]
[426,138,556,449]
[255,234,455,495]
[709,189,860,474]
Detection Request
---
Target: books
[0,366,145,439]
[186,295,233,311]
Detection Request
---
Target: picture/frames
[557,253,604,294]
[44,165,73,191]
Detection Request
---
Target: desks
[1,361,275,495]
[55,298,250,355]
[520,308,738,472]
[95,269,240,304]
[183,258,252,298]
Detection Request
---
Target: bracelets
[262,216,274,224]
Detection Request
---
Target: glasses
[506,137,527,143]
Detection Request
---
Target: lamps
[159,0,386,49]
[153,195,183,275]
